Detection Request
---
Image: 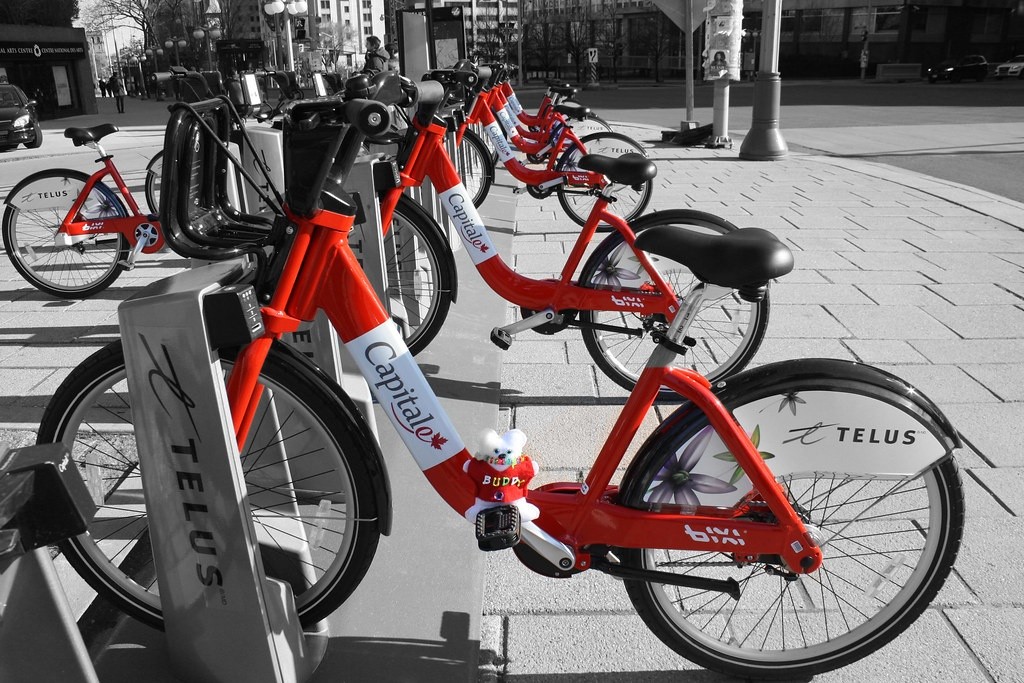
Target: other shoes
[118,110,126,114]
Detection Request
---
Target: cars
[993,54,1024,80]
[0,83,42,149]
[927,55,989,84]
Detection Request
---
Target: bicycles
[2,65,305,300]
[352,59,772,403]
[35,71,965,681]
[456,56,654,233]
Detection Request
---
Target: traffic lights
[295,29,306,40]
[861,30,867,41]
[294,18,306,29]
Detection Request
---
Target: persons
[711,51,726,66]
[110,72,125,113]
[99,80,112,98]
[361,36,390,72]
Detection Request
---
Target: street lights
[745,28,761,53]
[132,56,148,100]
[193,25,221,71]
[264,0,308,71]
[145,46,164,72]
[165,36,187,66]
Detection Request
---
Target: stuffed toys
[463,429,539,524]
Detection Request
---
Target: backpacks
[109,76,121,93]
